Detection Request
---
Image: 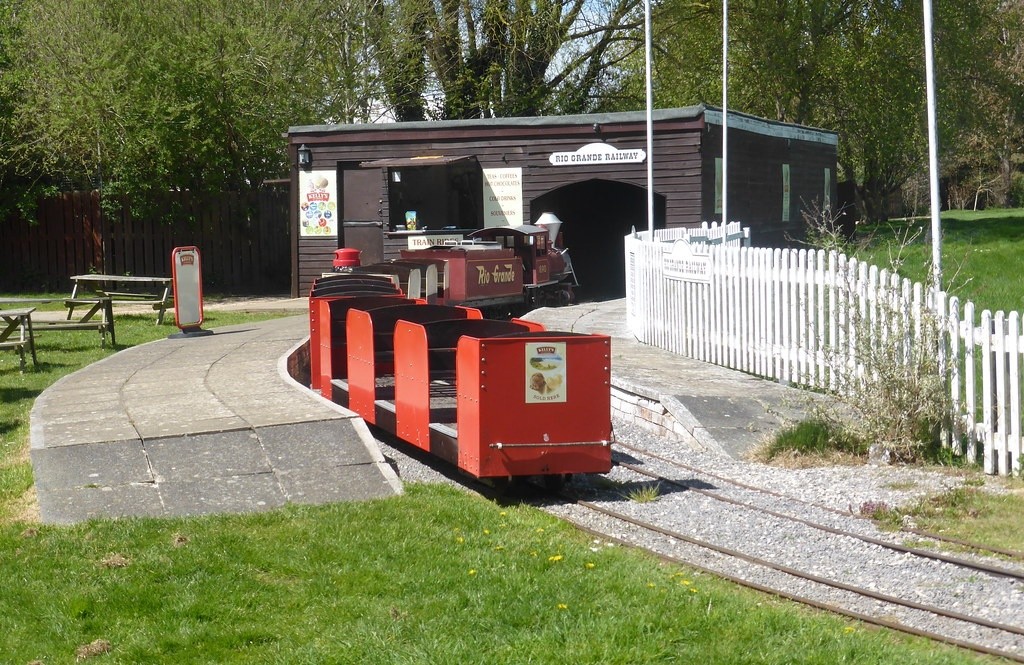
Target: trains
[308,224,615,501]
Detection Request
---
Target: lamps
[298,144,311,166]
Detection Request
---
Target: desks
[0,306,38,374]
[0,296,115,349]
[66,274,173,328]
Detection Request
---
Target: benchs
[0,334,41,374]
[1,320,116,350]
[62,292,174,326]
